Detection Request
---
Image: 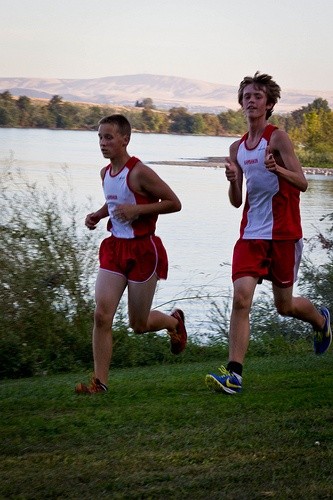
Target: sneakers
[75,376,108,396]
[168,309,187,354]
[205,365,242,394]
[314,306,332,355]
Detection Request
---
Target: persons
[75,113,188,395]
[205,69,333,396]
